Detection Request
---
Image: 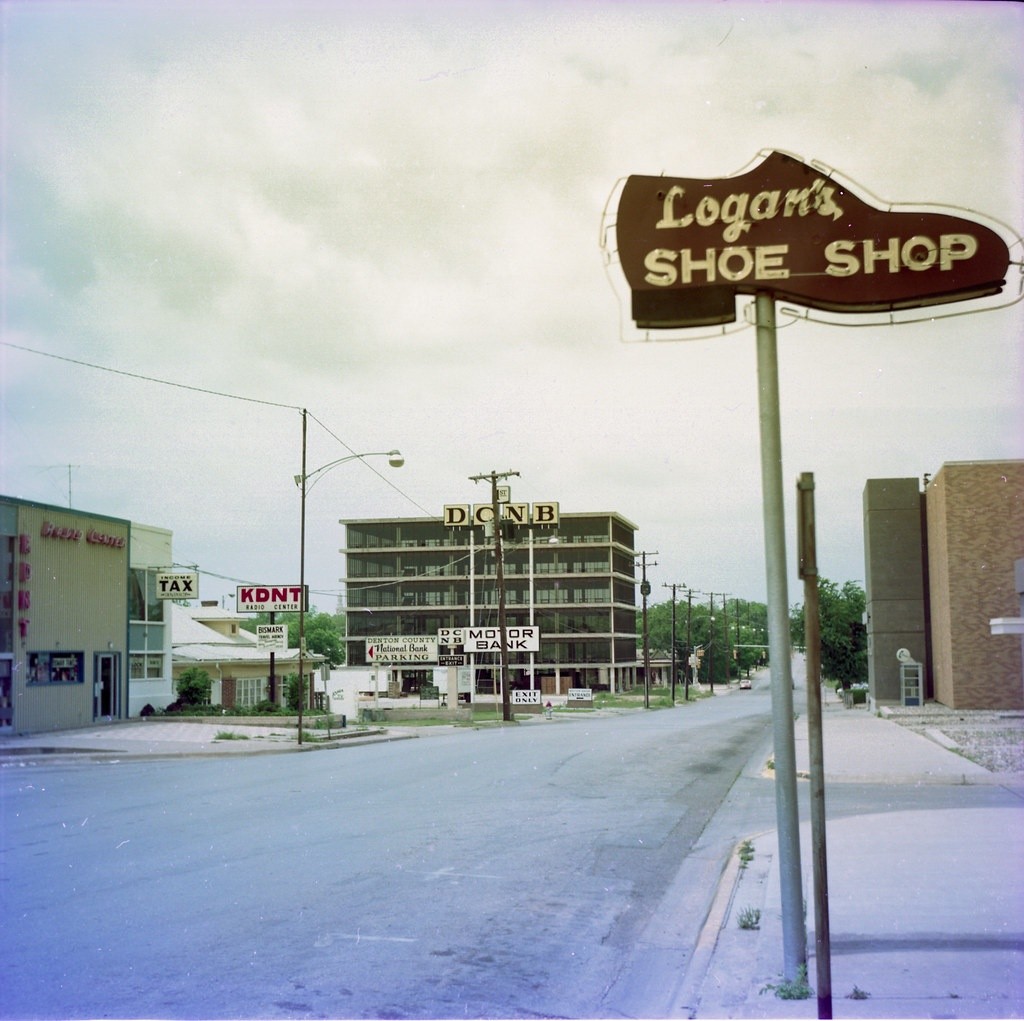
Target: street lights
[684,614,716,700]
[294,448,405,746]
[498,537,561,717]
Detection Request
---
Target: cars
[740,679,751,689]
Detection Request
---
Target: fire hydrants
[545,701,552,720]
